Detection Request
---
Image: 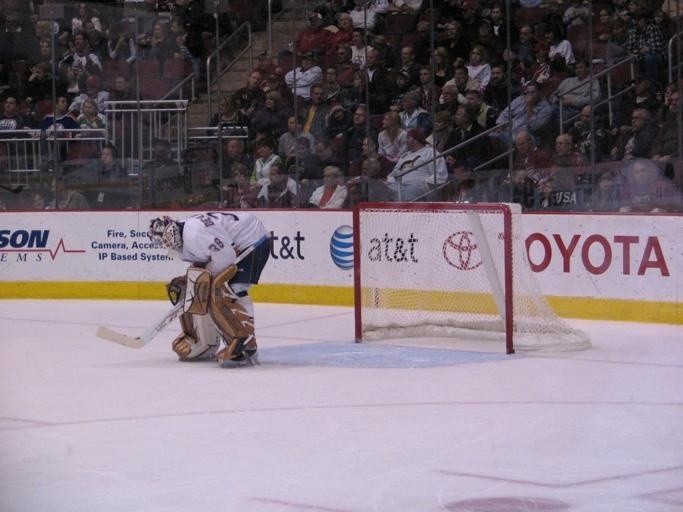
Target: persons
[1,1,682,213]
[150,213,270,367]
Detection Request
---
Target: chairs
[0,1,632,187]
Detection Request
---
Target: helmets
[147,216,183,253]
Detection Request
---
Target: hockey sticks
[97,232,271,349]
